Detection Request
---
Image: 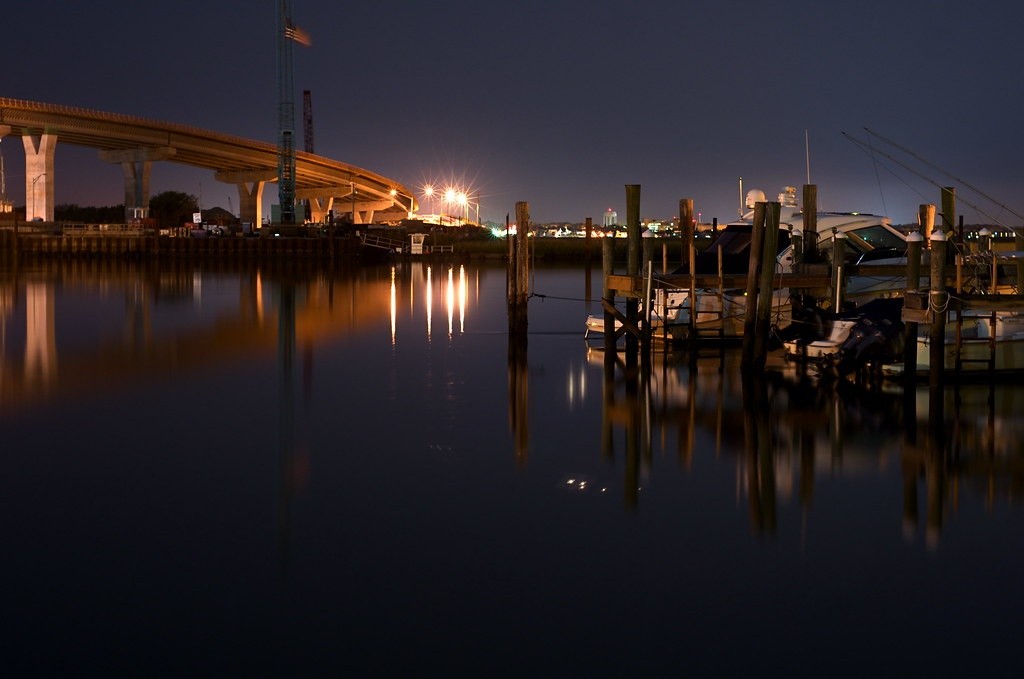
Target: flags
[285,18,312,48]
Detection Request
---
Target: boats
[584,126,1024,377]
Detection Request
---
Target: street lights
[391,189,414,221]
[422,185,480,228]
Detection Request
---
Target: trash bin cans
[243,223,254,236]
